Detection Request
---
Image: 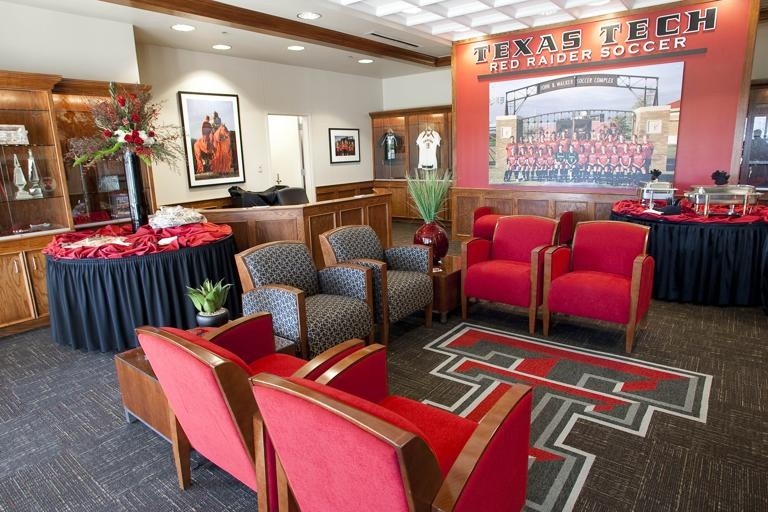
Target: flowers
[64,81,188,168]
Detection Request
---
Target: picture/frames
[329,128,361,163]
[178,90,245,188]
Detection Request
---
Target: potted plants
[184,278,234,328]
[650,169,662,182]
[399,169,460,264]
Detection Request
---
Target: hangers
[386,128,394,133]
[425,126,432,130]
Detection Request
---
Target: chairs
[234,240,375,360]
[319,225,434,350]
[541,220,655,353]
[136,312,366,512]
[248,344,532,512]
[274,187,309,205]
[462,215,560,334]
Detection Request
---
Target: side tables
[433,256,461,323]
[115,320,296,445]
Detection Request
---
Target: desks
[41,222,242,353]
[610,199,768,307]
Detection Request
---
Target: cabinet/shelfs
[1,71,76,337]
[369,104,452,222]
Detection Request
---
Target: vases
[121,148,146,231]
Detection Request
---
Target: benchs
[468,207,573,245]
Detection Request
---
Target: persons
[504,127,654,187]
[739,129,768,180]
[202,112,225,150]
